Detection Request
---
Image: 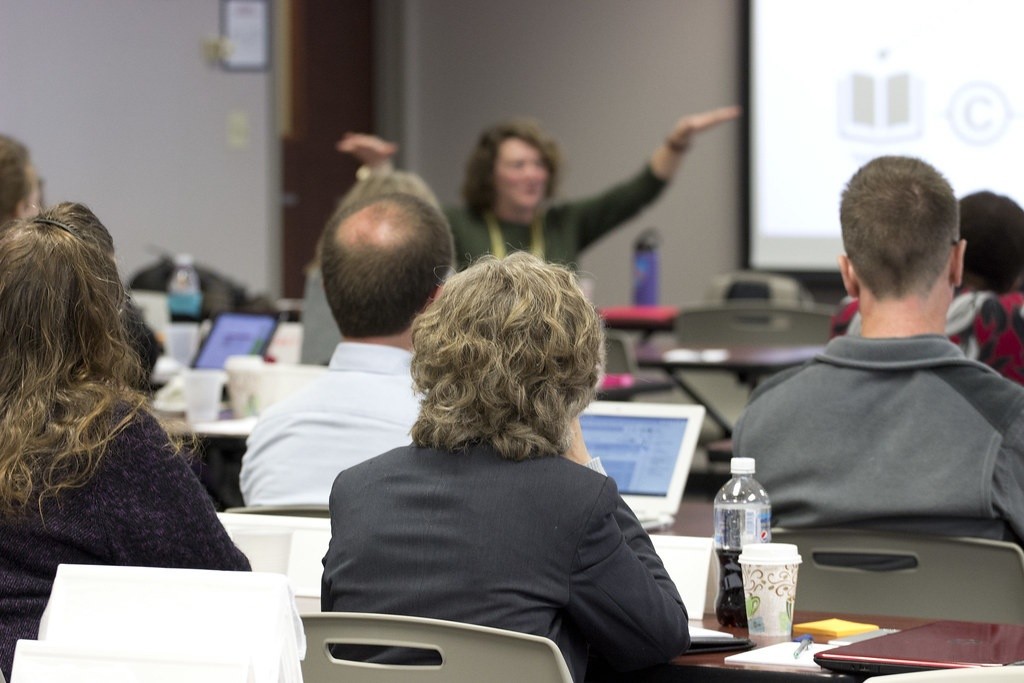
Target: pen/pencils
[792,634,813,659]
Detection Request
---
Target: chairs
[675,299,840,349]
[769,526,1024,628]
[299,613,574,683]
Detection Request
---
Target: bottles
[264,311,305,365]
[168,255,203,324]
[632,229,661,305]
[712,457,771,629]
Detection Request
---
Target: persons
[238,171,461,509]
[0,133,251,683]
[321,251,691,683]
[336,105,744,281]
[730,155,1024,556]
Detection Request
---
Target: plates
[151,403,185,418]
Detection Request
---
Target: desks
[165,414,261,513]
[652,611,1024,683]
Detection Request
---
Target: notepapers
[792,616,879,645]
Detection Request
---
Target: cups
[737,544,803,647]
[225,356,266,419]
[183,369,224,426]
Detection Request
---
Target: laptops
[814,620,1024,677]
[577,399,707,531]
[188,309,280,369]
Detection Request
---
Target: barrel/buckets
[577,270,601,306]
